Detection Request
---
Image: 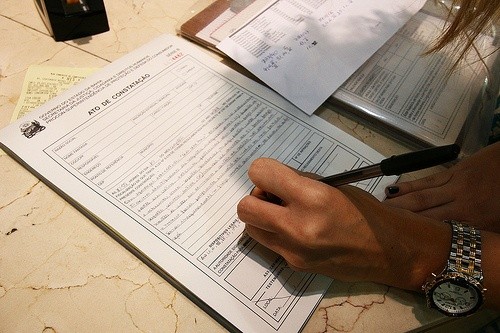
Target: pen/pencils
[266,144,461,205]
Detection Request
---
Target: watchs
[423,220,489,318]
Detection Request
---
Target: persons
[235,0,500,319]
